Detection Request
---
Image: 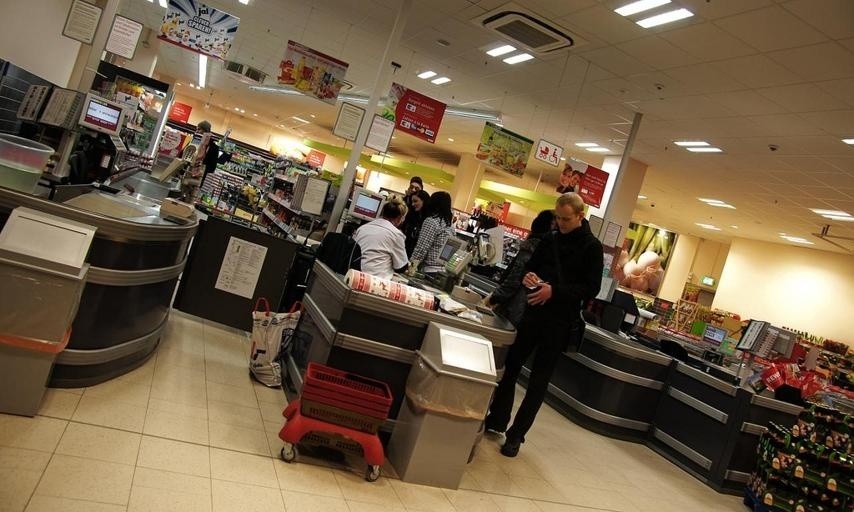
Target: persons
[350,177,454,281]
[475,192,604,458]
[555,164,583,193]
[195,120,219,186]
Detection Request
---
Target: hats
[410,176,423,191]
[199,121,211,132]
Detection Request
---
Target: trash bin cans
[385,321,498,489]
[0,206,96,417]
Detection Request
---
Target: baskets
[299,360,393,459]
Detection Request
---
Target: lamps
[250,51,508,124]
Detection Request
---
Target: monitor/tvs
[347,186,386,222]
[435,235,468,266]
[702,323,729,347]
[770,325,797,358]
[78,92,128,137]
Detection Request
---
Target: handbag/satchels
[248,309,301,388]
[565,310,586,353]
[419,264,447,284]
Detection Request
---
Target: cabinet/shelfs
[257,173,340,249]
[666,298,854,417]
[92,58,275,224]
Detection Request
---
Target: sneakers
[485,416,520,457]
